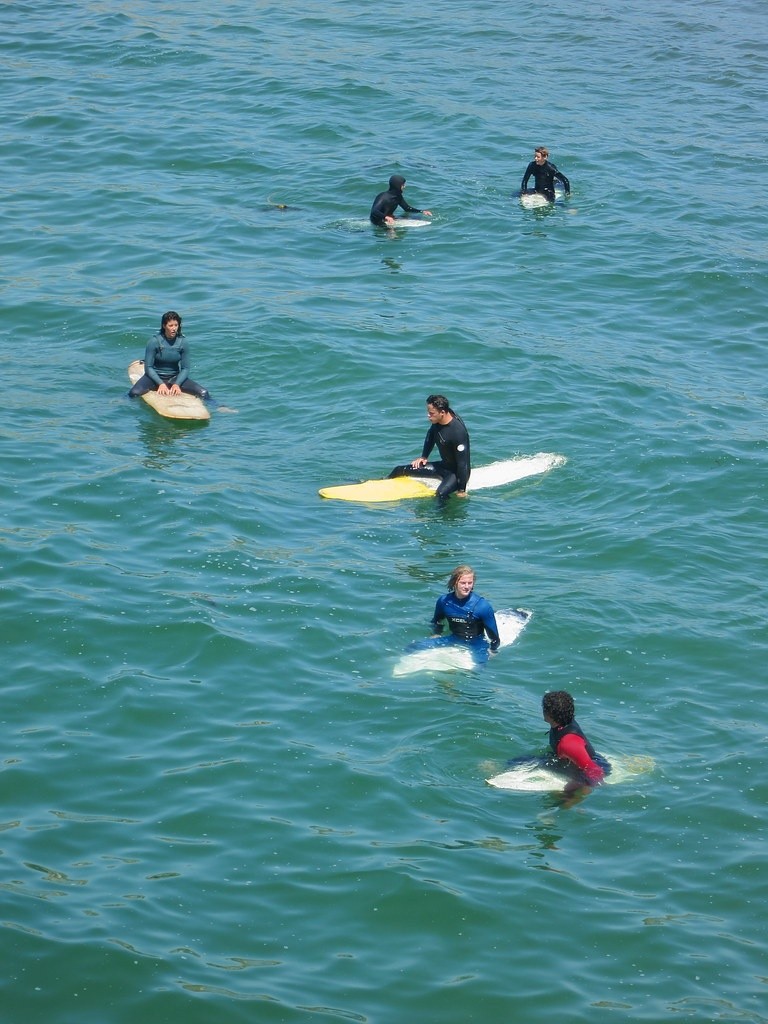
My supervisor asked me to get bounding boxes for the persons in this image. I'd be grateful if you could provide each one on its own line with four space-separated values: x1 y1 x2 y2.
513 146 576 214
370 175 432 238
403 565 501 670
360 395 470 504
127 312 239 414
480 692 612 827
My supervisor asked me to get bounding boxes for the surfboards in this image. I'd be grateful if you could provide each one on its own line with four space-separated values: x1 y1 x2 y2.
128 359 211 421
318 451 567 503
520 190 561 210
385 218 432 229
393 607 532 680
484 754 656 793
